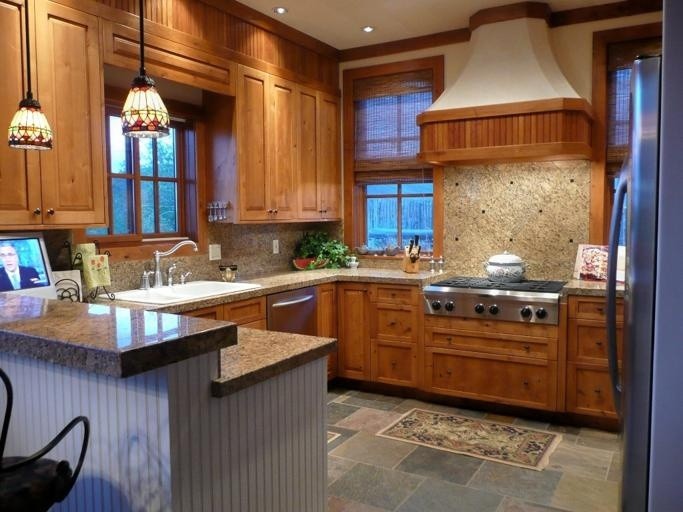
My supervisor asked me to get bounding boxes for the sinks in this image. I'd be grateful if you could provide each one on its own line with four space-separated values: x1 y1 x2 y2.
99 280 261 305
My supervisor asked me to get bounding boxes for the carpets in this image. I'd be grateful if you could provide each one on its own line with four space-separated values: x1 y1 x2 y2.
375 407 563 472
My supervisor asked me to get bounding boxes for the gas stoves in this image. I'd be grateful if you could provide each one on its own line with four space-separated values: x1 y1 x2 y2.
424 277 567 326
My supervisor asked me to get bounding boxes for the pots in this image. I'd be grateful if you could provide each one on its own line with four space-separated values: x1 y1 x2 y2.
482 249 526 284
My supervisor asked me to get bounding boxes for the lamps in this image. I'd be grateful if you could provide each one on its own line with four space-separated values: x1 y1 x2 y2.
120 0 169 138
7 0 52 150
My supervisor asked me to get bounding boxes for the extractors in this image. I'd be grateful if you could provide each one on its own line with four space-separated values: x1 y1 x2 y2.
415 5 597 169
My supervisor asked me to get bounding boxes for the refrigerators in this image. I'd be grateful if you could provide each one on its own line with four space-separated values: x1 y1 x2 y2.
607 0 683 511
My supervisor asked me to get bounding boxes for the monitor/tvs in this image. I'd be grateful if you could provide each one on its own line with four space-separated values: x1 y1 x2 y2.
0 232 58 300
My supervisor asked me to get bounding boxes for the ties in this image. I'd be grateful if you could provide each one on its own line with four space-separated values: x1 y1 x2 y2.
11 272 21 291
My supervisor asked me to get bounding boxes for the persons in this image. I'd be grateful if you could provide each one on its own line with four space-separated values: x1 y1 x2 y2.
1 244 42 289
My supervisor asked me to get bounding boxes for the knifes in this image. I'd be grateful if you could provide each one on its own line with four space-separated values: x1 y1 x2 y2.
414 235 418 248
416 246 421 256
408 239 414 254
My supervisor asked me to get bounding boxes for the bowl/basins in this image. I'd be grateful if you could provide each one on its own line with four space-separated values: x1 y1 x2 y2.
368 251 376 255
376 251 386 256
350 262 359 268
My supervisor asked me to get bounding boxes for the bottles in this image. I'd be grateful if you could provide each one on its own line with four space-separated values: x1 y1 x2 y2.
429 255 444 273
350 254 357 262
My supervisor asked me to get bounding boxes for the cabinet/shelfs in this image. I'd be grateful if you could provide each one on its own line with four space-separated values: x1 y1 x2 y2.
337 283 624 430
2 1 111 229
177 282 335 381
204 63 342 225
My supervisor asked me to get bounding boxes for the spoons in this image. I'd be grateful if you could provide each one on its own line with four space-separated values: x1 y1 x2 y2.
208 202 227 223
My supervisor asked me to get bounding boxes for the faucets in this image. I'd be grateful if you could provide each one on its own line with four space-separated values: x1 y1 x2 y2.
152 240 198 287
